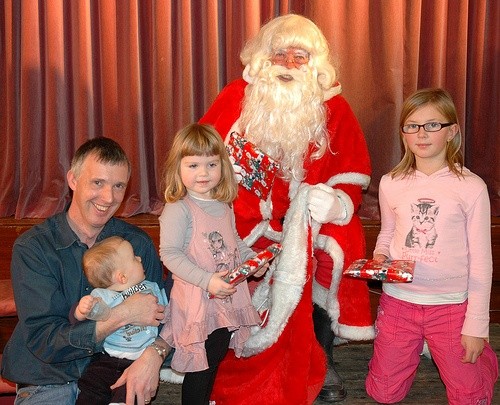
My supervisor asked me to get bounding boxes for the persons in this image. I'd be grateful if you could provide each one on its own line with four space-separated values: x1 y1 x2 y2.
181 11 376 405
0 135 187 405
364 86 499 404
66 237 173 405
156 121 271 405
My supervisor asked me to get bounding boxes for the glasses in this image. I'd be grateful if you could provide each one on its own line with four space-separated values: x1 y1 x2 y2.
401 121 455 134
271 51 308 64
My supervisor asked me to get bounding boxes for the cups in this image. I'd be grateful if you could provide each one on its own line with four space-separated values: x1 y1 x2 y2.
85 297 111 322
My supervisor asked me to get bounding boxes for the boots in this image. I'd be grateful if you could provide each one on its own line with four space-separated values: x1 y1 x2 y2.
312 302 347 402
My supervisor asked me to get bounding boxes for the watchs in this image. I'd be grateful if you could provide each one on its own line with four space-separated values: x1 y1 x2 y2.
150 342 167 361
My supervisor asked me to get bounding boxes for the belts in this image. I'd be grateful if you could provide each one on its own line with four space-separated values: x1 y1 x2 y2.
17 384 37 390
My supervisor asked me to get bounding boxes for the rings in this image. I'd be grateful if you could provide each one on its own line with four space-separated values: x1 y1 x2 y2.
145 399 151 402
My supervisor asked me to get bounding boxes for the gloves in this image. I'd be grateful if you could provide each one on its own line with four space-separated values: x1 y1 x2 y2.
306 182 347 224
